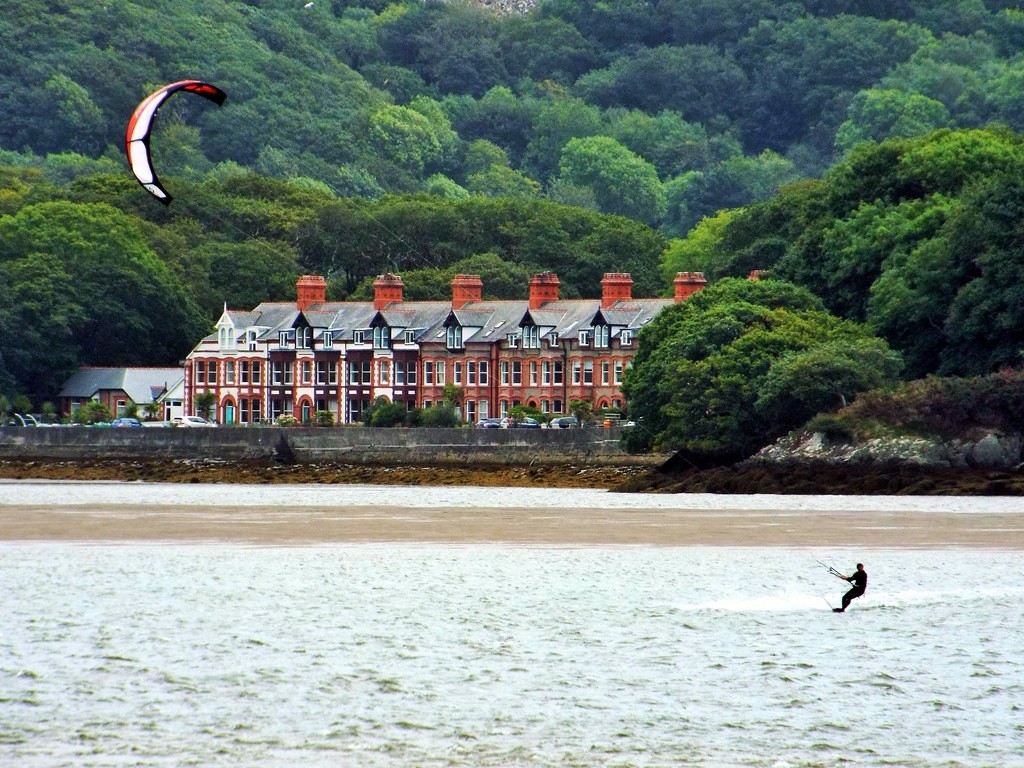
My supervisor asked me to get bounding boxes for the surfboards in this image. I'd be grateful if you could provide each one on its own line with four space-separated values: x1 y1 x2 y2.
833 608 843 612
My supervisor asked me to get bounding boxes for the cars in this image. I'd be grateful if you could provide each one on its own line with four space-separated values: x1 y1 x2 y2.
475 417 542 428
111 418 146 428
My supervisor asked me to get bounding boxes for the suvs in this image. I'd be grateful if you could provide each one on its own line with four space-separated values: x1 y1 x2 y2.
172 416 218 428
549 416 576 428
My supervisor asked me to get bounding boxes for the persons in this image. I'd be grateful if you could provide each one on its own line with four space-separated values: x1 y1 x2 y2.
833 563 868 612
